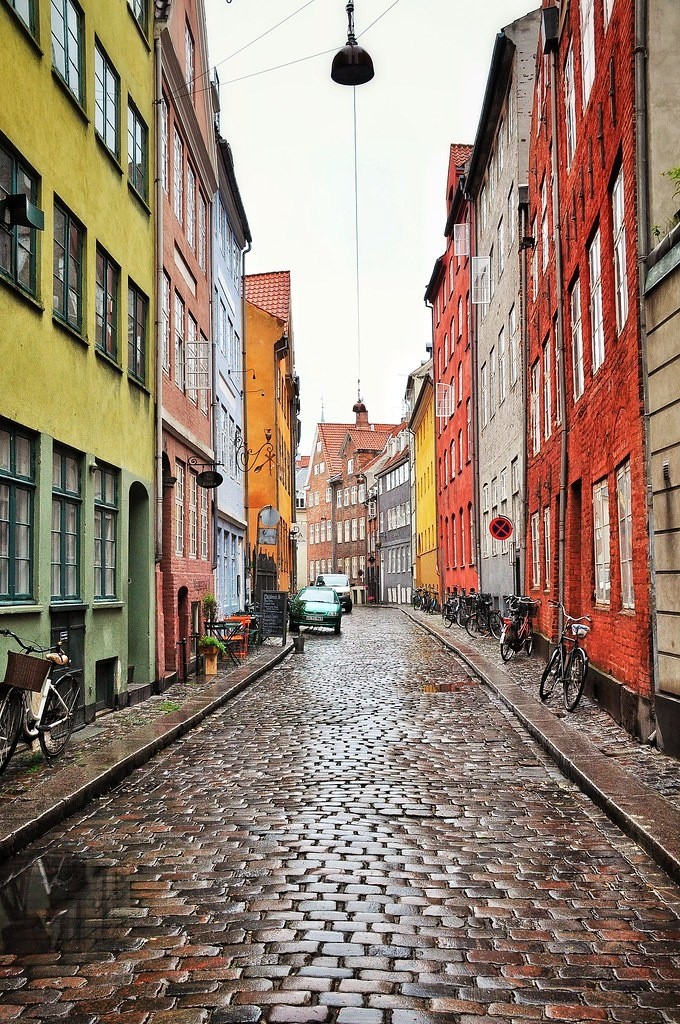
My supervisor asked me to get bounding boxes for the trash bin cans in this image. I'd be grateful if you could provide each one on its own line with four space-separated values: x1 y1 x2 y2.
293 637 304 653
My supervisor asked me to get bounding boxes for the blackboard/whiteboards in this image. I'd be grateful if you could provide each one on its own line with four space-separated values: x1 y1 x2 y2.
259 590 288 637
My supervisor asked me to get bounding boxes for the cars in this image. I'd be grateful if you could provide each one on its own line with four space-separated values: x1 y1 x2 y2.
290 585 345 635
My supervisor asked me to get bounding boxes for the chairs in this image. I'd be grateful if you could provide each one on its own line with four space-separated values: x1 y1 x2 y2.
213 610 265 659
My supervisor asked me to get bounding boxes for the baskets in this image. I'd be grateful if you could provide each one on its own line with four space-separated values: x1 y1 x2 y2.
465 597 473 606
520 606 538 617
3 650 52 693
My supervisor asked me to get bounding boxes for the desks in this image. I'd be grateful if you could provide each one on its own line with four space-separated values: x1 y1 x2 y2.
204 620 242 667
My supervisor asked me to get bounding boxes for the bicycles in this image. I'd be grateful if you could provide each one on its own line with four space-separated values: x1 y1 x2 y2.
0 629 84 778
412 589 542 662
540 599 594 711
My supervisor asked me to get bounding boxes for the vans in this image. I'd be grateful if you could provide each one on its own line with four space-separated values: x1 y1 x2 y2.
317 574 353 613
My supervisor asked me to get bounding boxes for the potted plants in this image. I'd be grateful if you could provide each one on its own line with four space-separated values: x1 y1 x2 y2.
658 166 680 221
198 593 226 676
290 597 306 653
